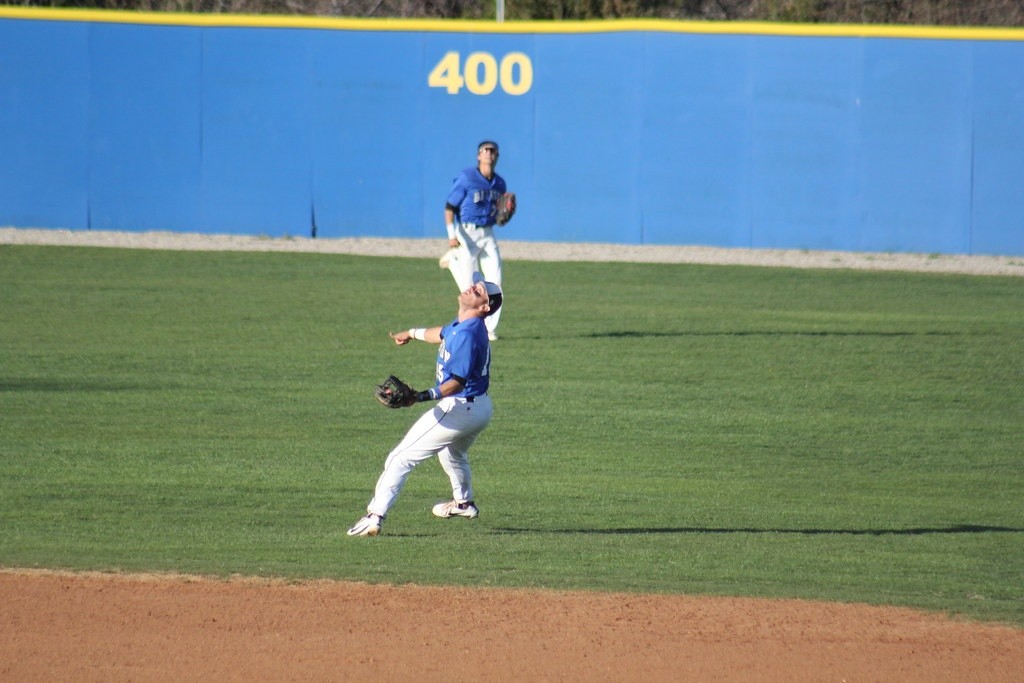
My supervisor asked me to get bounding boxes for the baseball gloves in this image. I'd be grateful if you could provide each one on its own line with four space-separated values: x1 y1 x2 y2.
375 374 419 409
495 192 516 226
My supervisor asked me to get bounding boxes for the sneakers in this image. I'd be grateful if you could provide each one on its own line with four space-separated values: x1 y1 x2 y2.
432 500 479 519
348 515 381 536
489 333 498 341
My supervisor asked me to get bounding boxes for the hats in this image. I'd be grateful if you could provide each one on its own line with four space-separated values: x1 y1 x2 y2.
473 271 502 316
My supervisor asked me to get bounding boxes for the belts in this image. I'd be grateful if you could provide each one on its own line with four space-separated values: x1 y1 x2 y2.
467 392 487 402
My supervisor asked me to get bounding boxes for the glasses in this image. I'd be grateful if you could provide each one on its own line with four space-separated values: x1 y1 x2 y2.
478 148 497 154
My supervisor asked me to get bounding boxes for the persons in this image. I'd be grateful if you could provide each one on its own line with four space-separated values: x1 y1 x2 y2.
444 140 516 341
348 280 504 538
